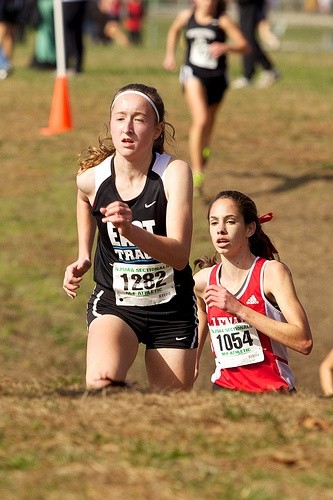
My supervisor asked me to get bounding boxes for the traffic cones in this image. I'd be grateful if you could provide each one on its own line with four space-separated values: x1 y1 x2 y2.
39 73 77 137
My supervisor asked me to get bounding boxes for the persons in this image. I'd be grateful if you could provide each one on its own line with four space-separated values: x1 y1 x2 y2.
62 84 200 393
319 349 333 398
192 190 312 393
163 0 250 190
1 0 280 90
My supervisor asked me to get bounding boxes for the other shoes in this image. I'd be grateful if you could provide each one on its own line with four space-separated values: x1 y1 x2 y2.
202 144 210 165
232 76 250 87
258 68 283 90
193 171 204 196
